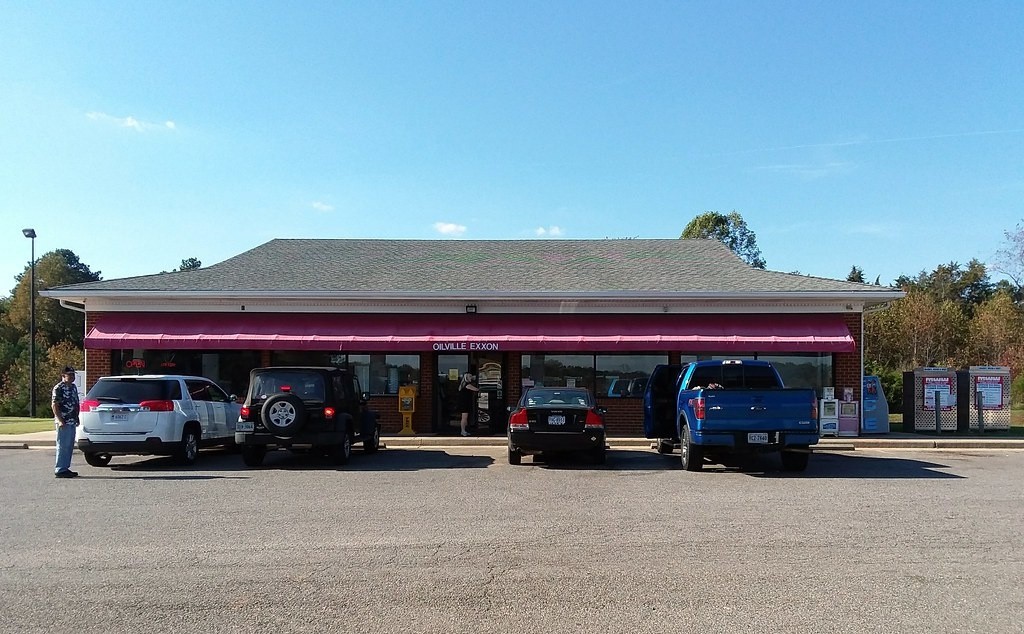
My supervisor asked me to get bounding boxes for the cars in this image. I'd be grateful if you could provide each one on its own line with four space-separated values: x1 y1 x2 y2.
506 386 609 465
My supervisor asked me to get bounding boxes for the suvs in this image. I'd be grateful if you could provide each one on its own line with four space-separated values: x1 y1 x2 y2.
76 372 243 467
234 366 380 468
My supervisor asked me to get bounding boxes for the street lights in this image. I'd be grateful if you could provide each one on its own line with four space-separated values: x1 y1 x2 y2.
22 228 37 418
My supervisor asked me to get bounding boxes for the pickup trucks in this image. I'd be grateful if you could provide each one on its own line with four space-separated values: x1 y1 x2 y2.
607 377 649 398
642 358 820 473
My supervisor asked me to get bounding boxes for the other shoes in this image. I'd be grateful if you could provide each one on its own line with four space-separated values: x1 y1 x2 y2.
461 431 471 436
55 469 78 478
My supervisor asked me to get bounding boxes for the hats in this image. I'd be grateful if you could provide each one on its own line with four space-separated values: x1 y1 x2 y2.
62 366 77 373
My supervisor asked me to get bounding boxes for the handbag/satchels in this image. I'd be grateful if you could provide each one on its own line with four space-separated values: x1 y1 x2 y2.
455 383 474 409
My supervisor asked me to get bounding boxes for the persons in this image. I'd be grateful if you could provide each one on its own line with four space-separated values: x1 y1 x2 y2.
52 366 80 478
458 374 479 436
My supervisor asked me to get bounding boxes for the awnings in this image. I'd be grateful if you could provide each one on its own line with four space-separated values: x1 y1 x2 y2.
84 312 855 351
